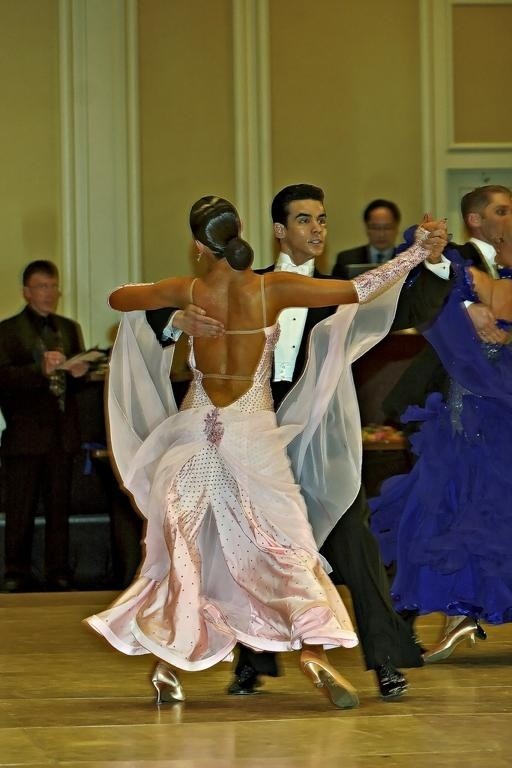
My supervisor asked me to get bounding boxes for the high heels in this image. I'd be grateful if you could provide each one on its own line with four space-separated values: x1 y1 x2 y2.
420 615 477 662
151 664 186 706
299 650 360 709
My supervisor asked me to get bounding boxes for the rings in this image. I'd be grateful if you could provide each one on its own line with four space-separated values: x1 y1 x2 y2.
56 359 60 365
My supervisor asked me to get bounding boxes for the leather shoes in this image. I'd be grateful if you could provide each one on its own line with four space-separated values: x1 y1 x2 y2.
228 666 260 694
375 655 410 698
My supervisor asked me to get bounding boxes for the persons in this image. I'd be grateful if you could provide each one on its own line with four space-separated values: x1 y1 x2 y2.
97 315 142 589
331 197 403 279
1 258 107 592
102 191 447 712
142 182 455 701
